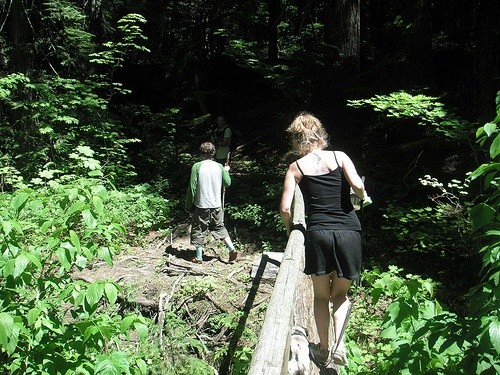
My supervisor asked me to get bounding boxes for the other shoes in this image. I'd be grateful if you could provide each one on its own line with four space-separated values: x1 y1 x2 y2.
192 256 203 264
229 249 237 261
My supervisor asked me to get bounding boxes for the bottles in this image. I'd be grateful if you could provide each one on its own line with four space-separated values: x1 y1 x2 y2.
349 186 361 210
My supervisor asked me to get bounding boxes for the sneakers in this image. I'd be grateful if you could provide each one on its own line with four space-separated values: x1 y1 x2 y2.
331 342 348 366
309 342 330 364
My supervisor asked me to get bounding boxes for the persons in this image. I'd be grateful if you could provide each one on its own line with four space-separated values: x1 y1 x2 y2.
278 114 367 366
185 142 237 263
